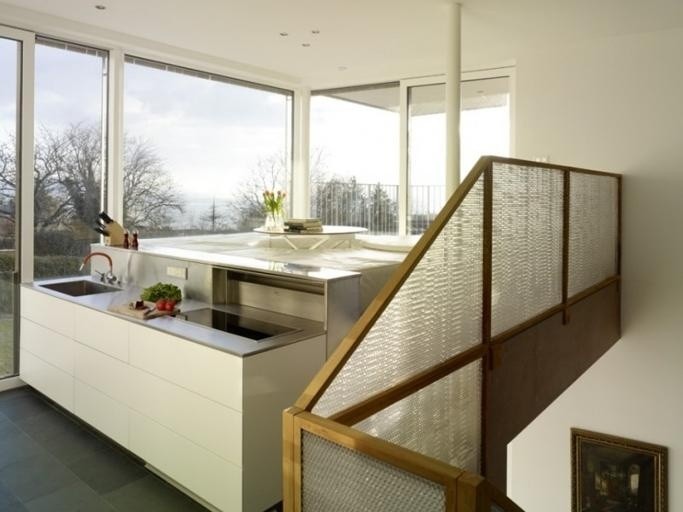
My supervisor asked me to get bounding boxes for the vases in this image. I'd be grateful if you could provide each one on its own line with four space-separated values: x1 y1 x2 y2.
264 212 284 233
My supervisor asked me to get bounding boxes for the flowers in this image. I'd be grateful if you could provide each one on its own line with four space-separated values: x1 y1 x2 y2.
263 191 286 222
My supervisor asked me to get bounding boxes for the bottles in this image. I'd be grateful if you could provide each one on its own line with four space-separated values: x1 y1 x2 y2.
121 227 140 251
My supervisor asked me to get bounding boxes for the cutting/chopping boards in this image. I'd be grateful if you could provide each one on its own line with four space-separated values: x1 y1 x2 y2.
106 302 178 321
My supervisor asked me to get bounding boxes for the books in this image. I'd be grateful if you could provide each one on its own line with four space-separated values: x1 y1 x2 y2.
283 218 324 234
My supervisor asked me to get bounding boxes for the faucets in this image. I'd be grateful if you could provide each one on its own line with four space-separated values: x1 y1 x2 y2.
78 253 116 285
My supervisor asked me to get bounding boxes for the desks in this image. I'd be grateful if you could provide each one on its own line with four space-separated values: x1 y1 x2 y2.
253 225 368 250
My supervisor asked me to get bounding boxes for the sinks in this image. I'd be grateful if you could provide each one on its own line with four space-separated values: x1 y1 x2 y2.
40 279 122 297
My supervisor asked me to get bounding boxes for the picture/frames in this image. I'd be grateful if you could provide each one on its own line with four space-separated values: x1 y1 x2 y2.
570 428 667 512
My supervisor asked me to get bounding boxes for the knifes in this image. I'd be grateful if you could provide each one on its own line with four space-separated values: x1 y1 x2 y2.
143 306 156 316
93 210 114 237
